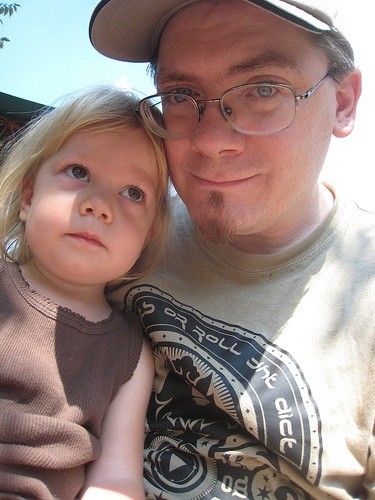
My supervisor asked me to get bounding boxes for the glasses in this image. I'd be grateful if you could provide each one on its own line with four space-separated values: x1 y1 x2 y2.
135 64 340 140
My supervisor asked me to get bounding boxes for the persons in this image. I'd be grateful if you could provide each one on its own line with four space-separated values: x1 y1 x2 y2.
89 0 375 500
1 86 174 499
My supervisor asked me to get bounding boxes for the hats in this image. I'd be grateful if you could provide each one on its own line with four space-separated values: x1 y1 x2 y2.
88 0 356 62
